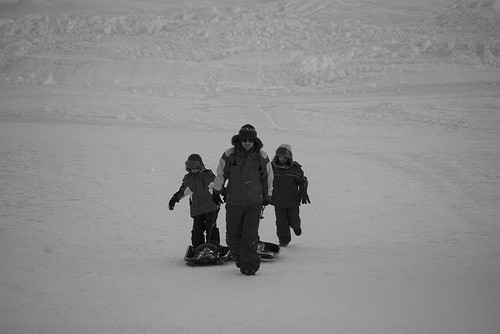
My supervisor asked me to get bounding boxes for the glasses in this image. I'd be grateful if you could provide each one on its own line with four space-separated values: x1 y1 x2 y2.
242 138 254 143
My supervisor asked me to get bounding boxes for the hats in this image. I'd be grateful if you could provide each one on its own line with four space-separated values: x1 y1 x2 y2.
275 144 293 164
185 154 205 174
239 124 257 138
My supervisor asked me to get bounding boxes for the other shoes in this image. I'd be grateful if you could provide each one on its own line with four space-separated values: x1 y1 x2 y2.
293 223 301 236
279 240 288 246
235 255 255 275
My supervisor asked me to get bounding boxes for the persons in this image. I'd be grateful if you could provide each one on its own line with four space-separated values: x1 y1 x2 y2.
211 124 274 275
169 154 226 257
269 144 312 247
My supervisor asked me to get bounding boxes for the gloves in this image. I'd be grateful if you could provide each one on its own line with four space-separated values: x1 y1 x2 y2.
212 190 223 205
222 191 227 202
262 196 270 205
298 191 310 204
169 197 177 210
